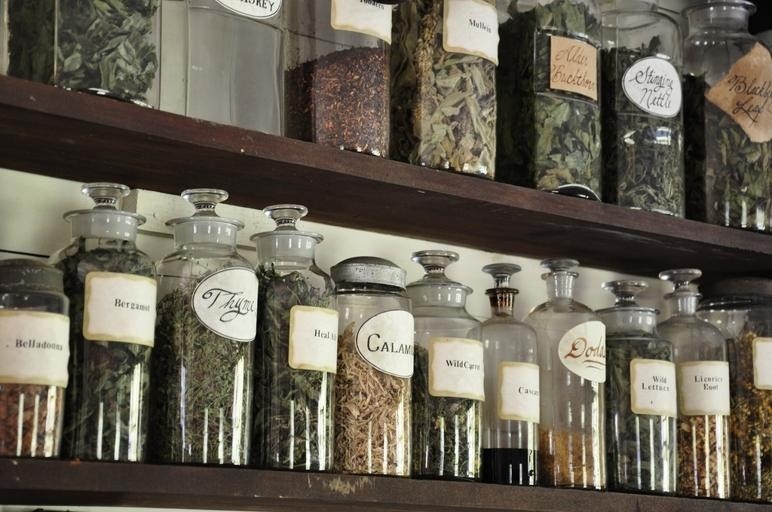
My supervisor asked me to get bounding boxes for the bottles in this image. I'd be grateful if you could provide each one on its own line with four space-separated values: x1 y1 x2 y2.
518 258 607 492
184 1 290 138
148 188 259 468
497 1 601 200
404 250 482 480
690 291 772 503
390 2 500 181
282 0 395 161
0 257 70 459
677 1 771 235
248 203 337 474
0 0 168 111
330 255 414 480
655 267 733 502
48 182 160 462
466 262 540 487
597 1 688 221
594 277 681 497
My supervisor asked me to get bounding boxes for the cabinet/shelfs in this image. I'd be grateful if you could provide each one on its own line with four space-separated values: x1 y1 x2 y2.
0 74 772 512
